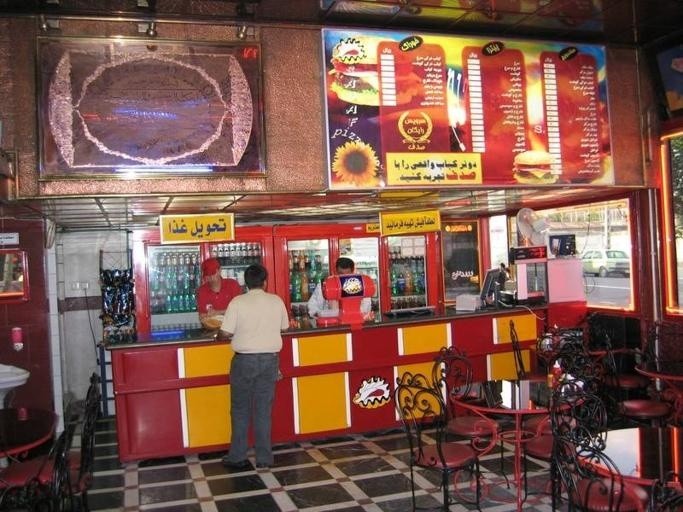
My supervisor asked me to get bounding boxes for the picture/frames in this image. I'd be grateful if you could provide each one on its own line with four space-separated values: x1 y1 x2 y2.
33 34 267 183
643 31 682 122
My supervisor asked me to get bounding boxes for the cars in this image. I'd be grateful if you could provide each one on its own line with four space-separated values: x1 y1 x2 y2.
582 249 630 278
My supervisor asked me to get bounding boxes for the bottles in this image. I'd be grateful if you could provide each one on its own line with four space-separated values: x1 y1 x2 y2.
391 269 425 296
151 322 203 342
211 243 262 265
389 247 424 268
153 251 200 266
356 270 378 297
392 297 426 308
371 299 380 322
289 248 323 271
290 272 325 302
292 304 310 330
149 265 201 290
150 290 198 313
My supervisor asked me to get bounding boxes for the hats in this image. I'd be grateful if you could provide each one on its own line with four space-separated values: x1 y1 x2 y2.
201 258 219 278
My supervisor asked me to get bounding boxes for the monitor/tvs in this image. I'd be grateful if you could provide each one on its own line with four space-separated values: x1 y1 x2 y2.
480 268 504 310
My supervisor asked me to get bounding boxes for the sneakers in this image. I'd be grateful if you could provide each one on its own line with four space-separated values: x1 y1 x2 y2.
222 457 249 468
256 462 271 472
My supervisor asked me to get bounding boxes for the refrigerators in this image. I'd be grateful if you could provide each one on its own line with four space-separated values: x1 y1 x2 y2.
134 225 275 342
383 223 439 318
279 220 383 331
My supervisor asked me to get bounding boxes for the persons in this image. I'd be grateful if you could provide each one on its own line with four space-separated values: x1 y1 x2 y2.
219 264 289 473
307 256 371 320
197 259 242 327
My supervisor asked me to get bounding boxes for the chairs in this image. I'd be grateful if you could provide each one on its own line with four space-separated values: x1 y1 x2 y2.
0 374 107 512
392 312 682 512
